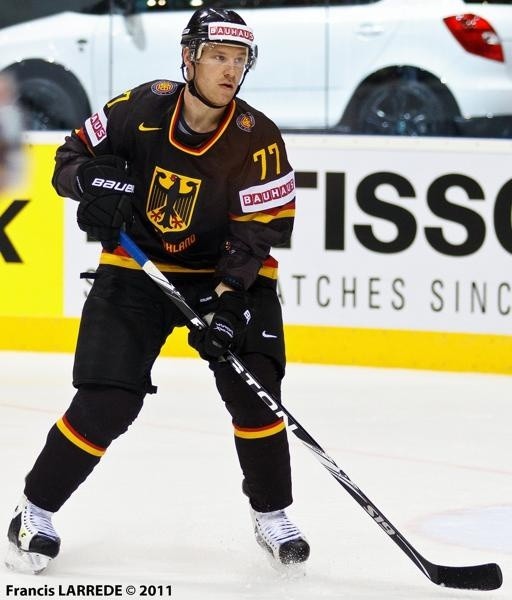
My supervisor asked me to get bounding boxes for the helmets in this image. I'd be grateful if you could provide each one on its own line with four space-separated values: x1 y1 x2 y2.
180 7 256 64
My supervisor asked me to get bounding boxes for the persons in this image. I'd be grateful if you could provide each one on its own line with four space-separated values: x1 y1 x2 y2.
1 6 312 574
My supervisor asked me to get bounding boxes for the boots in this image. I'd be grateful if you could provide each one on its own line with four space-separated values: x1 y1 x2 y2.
252 508 310 564
7 495 61 559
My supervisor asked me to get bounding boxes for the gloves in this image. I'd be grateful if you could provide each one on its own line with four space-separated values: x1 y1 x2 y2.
188 290 252 371
76 154 133 241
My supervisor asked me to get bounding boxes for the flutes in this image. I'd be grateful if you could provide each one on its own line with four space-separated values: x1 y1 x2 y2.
116 230 502 590
